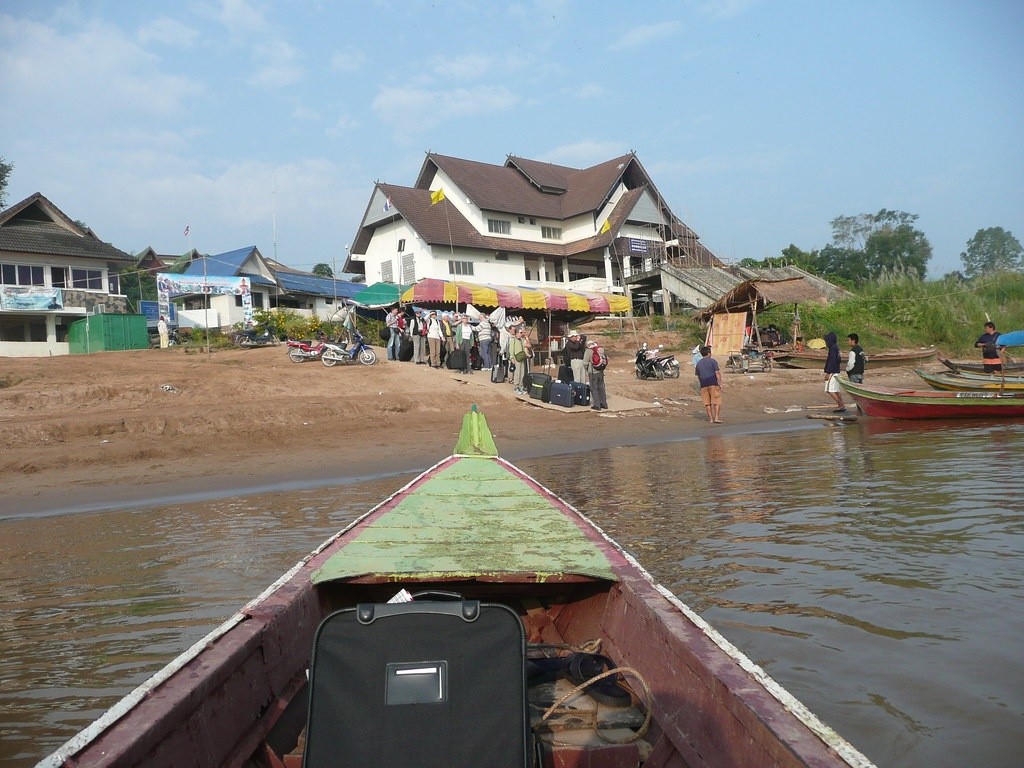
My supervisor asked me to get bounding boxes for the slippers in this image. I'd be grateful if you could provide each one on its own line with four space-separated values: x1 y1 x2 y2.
560 651 632 709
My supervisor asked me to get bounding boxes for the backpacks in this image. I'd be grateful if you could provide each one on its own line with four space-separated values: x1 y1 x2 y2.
591 346 608 370
542 380 553 404
550 381 576 407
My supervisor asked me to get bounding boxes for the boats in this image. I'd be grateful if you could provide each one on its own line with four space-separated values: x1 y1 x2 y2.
913 367 1024 392
935 354 1024 375
834 374 1024 421
772 344 939 371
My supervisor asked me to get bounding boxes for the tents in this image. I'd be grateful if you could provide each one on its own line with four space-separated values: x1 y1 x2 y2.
346 277 640 366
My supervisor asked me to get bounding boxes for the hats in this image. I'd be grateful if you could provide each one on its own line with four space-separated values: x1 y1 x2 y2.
566 330 580 337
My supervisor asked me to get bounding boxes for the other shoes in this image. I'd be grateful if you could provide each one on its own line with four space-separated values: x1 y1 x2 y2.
600 403 608 408
481 367 491 371
590 406 600 410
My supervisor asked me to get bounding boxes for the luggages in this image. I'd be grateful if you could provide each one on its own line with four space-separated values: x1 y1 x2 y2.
446 349 467 369
490 353 505 383
398 341 416 362
569 381 591 406
557 365 574 384
301 589 534 768
523 358 536 393
530 357 551 402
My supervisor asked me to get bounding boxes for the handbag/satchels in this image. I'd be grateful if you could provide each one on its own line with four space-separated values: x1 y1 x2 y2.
379 327 391 341
514 351 526 362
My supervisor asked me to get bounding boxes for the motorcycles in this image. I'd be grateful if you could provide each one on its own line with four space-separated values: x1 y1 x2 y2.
285 325 336 364
320 326 377 367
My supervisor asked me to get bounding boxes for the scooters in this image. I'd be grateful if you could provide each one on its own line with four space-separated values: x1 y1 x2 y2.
237 319 281 351
634 341 681 380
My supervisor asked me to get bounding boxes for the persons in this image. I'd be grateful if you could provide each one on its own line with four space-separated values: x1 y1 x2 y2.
502 316 534 394
48 297 61 309
823 332 847 412
410 309 500 374
695 346 724 423
845 333 864 416
567 329 587 384
158 316 169 348
385 306 407 360
750 323 786 347
975 322 1005 374
583 340 608 410
562 338 571 368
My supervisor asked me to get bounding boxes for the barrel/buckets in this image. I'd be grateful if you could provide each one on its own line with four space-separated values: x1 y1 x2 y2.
561 336 568 350
551 339 558 351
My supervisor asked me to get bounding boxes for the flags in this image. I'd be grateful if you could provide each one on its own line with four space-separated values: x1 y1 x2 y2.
184 225 189 236
601 220 611 236
383 199 390 212
431 188 444 204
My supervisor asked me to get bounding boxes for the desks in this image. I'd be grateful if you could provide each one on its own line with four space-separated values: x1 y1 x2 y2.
534 350 563 366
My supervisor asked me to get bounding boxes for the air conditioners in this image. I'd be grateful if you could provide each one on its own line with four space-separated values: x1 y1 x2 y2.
93 304 105 314
109 283 114 291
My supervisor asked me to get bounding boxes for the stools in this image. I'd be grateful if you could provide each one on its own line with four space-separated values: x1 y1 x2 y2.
556 356 565 366
